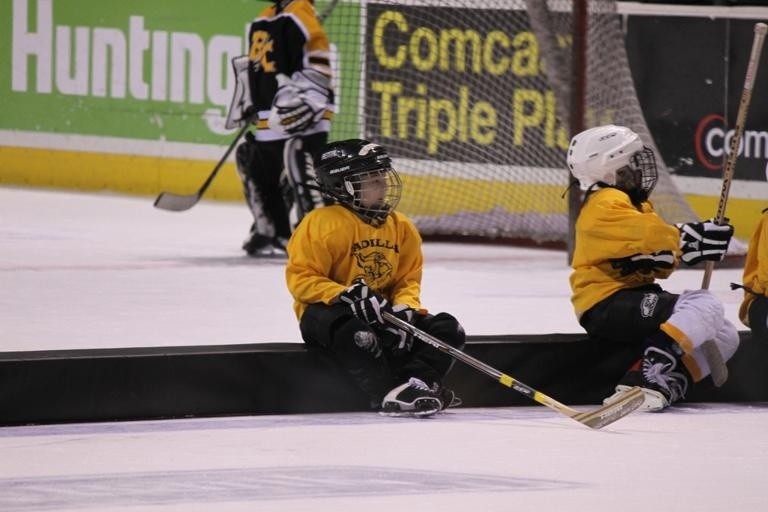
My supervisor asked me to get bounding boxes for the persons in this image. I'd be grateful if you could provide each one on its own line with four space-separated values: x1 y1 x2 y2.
236 0 335 257
739 208 768 332
285 139 467 416
568 123 740 412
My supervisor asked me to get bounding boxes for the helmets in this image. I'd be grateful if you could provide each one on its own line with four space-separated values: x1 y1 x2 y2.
566 125 658 204
313 140 404 227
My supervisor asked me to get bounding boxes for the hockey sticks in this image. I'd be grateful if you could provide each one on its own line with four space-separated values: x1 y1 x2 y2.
381 311 644 428
154 0 337 212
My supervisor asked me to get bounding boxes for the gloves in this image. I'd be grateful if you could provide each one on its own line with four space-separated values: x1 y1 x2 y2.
674 218 734 264
272 67 332 134
335 275 415 358
610 250 674 279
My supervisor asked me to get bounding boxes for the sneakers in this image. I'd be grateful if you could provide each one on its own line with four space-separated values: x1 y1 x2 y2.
243 233 294 258
616 346 695 406
380 369 455 418
